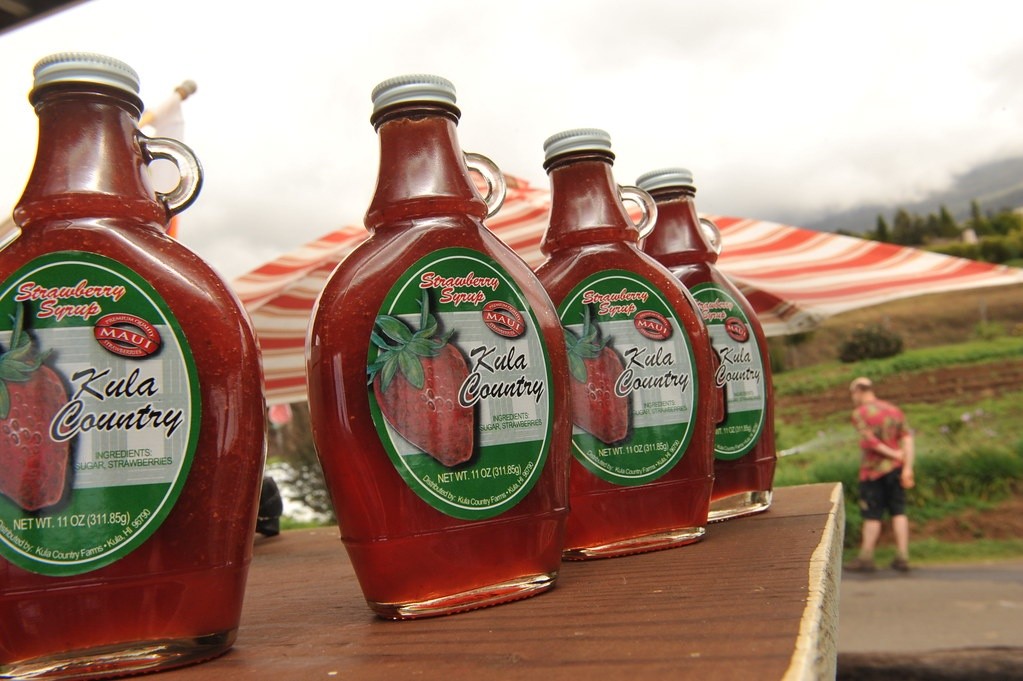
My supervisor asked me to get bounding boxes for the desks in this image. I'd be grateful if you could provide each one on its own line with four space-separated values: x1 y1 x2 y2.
101 480 848 681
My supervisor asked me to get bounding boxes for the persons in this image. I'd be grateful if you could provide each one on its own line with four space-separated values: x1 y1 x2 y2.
844 378 915 573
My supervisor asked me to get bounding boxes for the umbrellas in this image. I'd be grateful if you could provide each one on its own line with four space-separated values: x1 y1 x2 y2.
227 170 1023 401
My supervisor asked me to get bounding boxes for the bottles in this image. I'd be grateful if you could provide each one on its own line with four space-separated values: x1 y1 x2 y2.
633 167 778 524
0 50 268 679
533 128 716 563
306 74 573 622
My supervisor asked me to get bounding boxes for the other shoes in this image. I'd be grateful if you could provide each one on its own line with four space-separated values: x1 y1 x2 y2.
890 559 912 574
842 560 875 572
